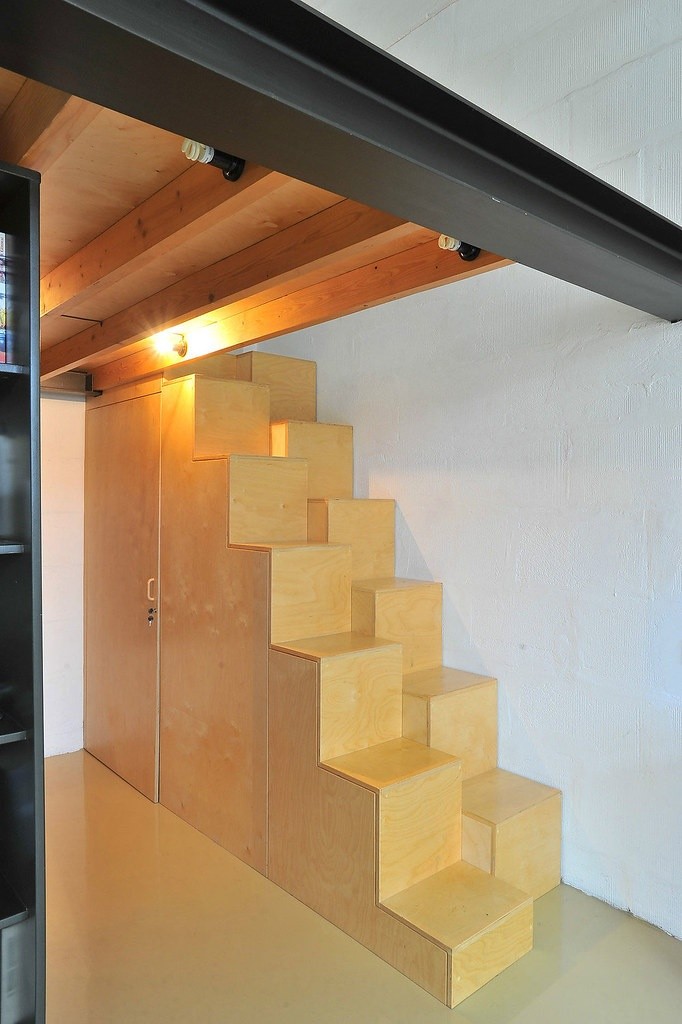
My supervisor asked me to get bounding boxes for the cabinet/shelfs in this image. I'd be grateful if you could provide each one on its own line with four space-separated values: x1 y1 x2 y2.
0 161 70 1024
